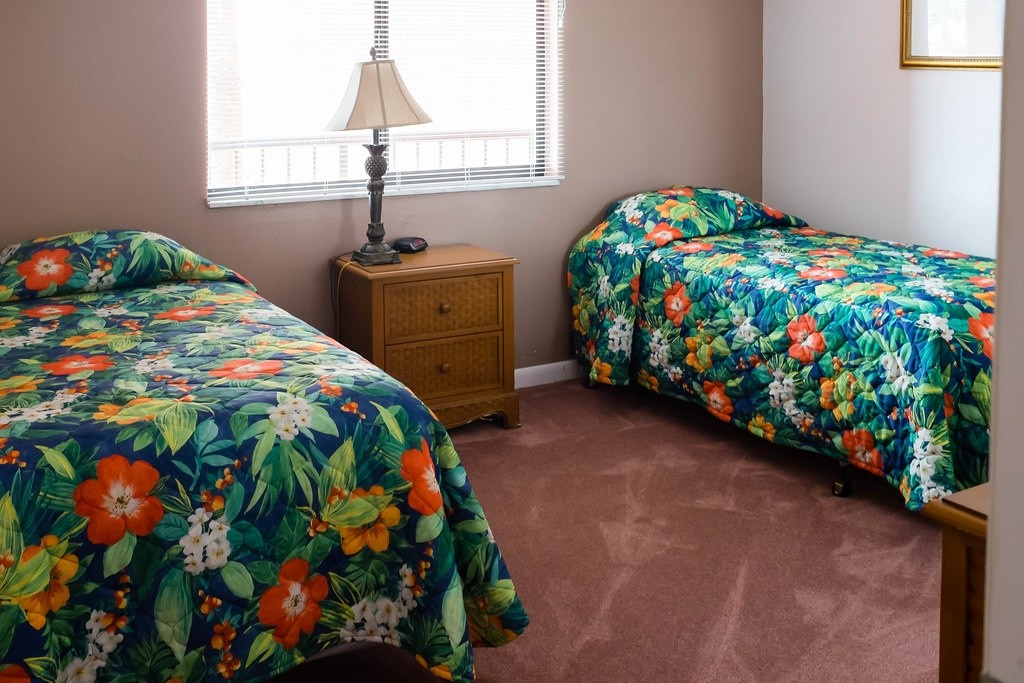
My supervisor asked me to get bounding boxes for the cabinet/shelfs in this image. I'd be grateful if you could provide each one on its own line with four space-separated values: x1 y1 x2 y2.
921 482 989 683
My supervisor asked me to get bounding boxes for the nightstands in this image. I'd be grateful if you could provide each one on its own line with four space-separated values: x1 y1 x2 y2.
329 242 522 430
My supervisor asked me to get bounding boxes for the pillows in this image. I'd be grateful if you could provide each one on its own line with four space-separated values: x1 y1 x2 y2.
0 229 259 305
603 186 785 249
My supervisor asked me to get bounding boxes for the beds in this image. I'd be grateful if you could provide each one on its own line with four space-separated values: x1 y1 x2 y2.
566 213 996 511
0 279 528 683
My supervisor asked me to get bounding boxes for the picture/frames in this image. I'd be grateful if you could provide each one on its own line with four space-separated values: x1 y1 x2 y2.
899 0 1006 72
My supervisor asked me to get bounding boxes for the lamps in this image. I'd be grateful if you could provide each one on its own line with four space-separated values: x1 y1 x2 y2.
324 46 434 267
